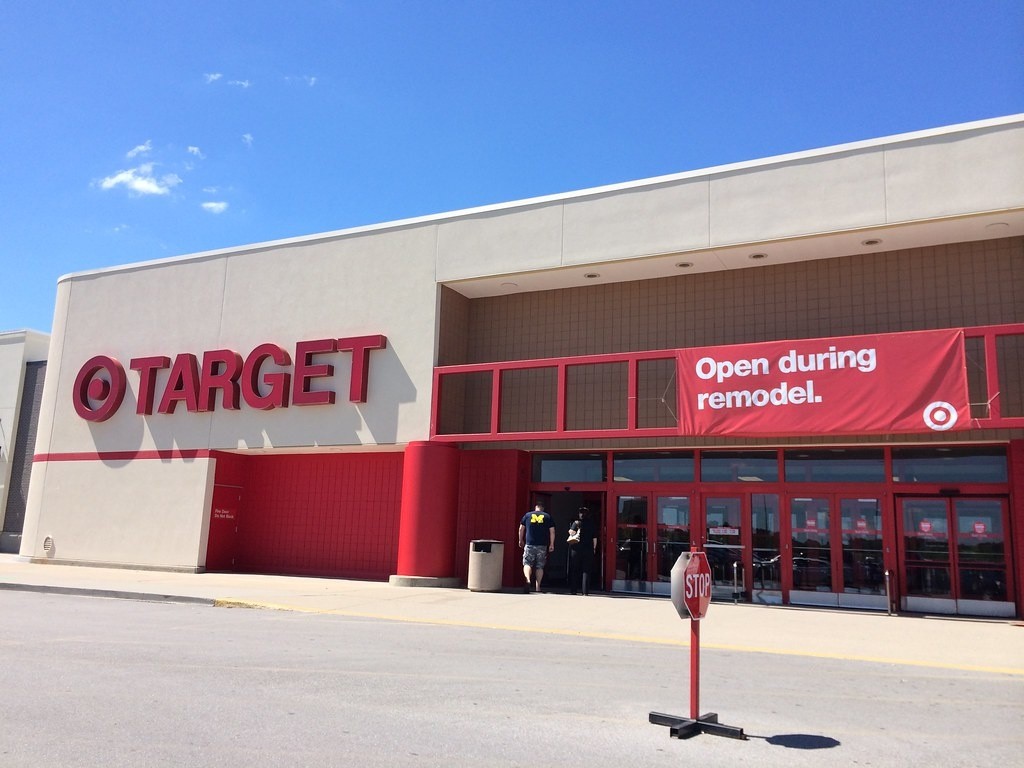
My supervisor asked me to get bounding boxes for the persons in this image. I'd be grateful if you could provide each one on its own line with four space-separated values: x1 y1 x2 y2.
518 502 555 592
568 505 597 596
624 515 647 578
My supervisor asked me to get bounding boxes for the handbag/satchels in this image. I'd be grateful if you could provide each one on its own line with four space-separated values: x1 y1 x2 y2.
567 521 581 545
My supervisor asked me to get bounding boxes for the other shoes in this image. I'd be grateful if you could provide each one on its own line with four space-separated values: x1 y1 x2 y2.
524 581 530 594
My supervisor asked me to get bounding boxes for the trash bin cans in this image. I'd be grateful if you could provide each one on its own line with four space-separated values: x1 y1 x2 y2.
466 539 505 592
